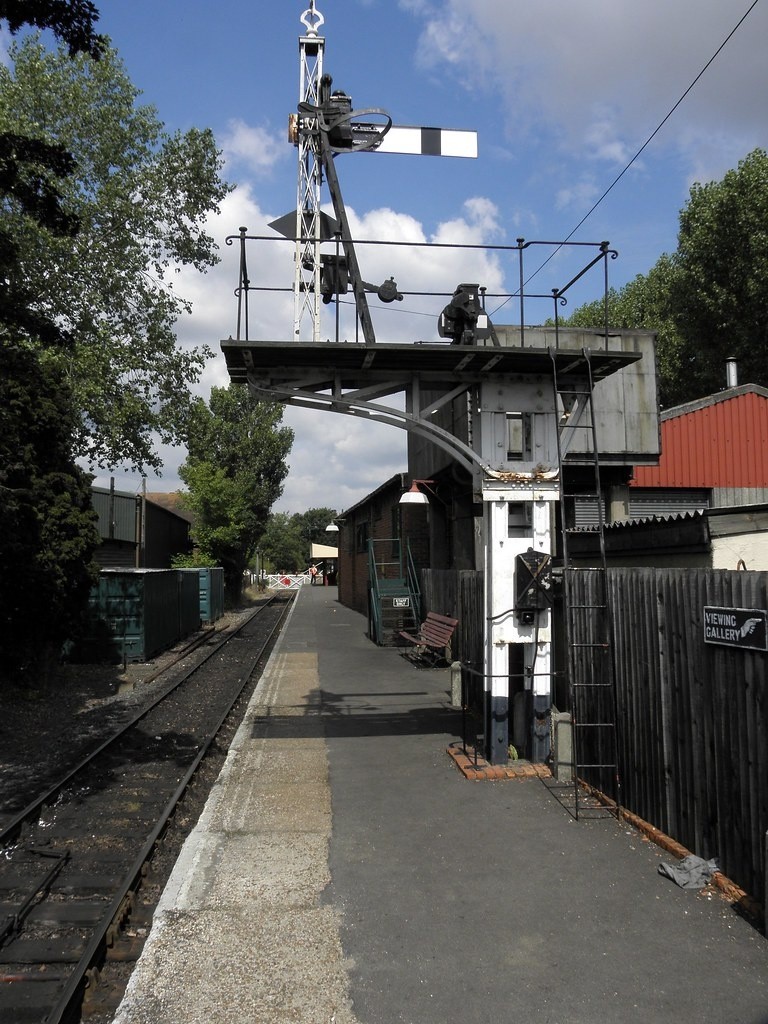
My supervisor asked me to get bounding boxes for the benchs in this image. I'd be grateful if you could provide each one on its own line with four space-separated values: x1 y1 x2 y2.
399 611 459 669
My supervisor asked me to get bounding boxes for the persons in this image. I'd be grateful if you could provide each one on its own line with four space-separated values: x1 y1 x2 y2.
310 564 317 584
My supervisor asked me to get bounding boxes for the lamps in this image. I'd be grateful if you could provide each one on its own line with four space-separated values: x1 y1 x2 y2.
399 477 436 504
